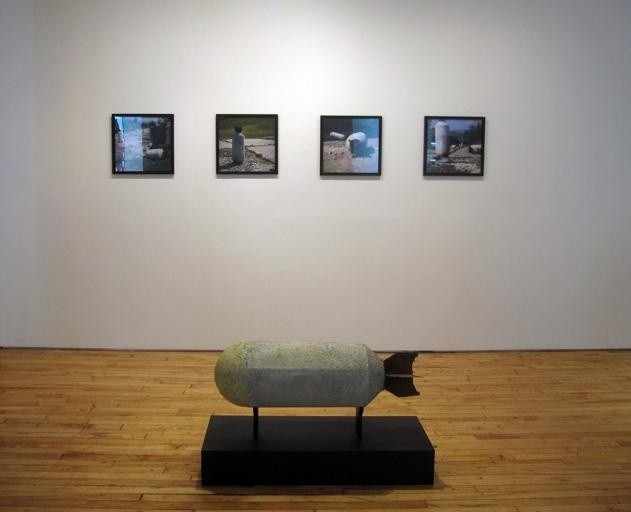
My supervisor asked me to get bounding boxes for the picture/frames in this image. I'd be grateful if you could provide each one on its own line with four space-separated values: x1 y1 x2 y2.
320 114 381 177
111 114 174 175
215 113 278 175
423 115 484 177
